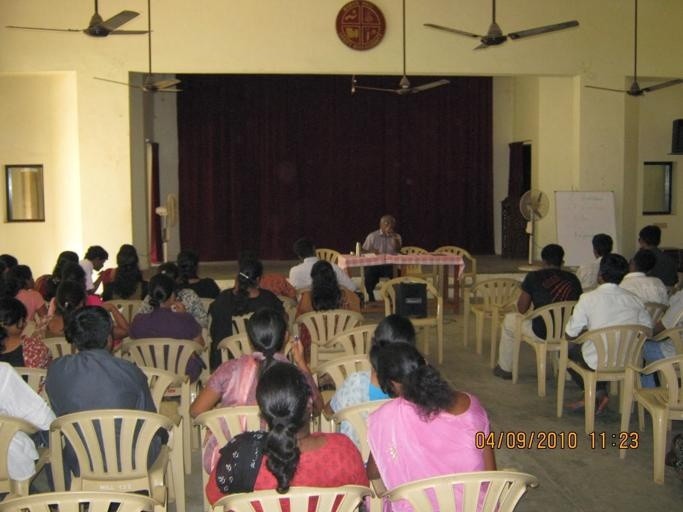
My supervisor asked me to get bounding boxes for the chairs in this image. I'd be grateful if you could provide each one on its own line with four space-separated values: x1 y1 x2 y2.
315 248 342 266
432 246 477 313
401 246 435 283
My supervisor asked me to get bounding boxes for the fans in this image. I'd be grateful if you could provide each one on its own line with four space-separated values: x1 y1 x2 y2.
6 0 153 37
352 0 451 95
584 0 683 95
155 193 177 264
423 0 579 50
519 189 549 271
93 0 184 93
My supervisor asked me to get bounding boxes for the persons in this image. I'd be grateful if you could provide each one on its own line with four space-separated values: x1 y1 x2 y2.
618 249 669 321
1 244 496 512
361 214 402 303
493 244 583 380
641 289 682 389
574 233 614 290
637 225 679 287
563 253 654 416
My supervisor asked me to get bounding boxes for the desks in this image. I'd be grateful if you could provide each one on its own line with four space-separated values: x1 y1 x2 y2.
337 252 465 313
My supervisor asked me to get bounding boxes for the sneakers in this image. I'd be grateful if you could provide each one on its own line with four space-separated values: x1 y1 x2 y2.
492 364 512 379
563 392 611 416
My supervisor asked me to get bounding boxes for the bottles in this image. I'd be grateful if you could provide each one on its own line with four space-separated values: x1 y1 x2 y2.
356 241 360 257
